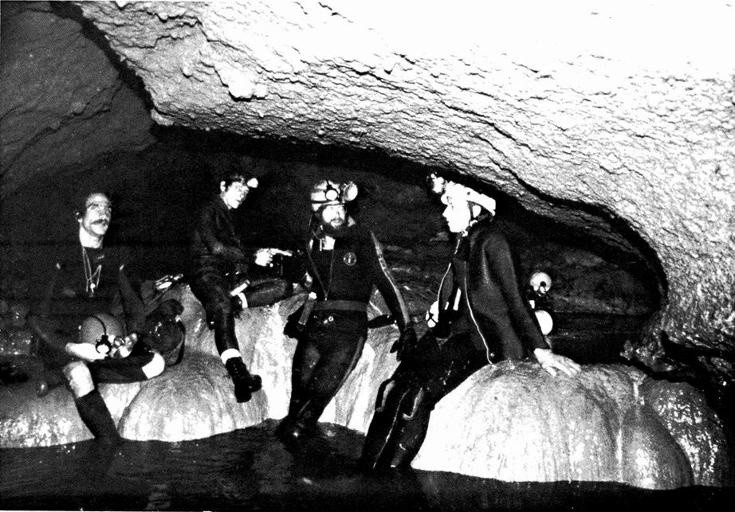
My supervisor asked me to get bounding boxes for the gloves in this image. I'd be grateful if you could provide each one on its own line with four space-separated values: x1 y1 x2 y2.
388 321 419 361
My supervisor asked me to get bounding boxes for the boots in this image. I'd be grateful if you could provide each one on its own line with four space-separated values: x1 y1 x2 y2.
225 353 265 404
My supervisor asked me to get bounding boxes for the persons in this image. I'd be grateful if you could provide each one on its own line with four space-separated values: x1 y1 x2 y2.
223 173 421 500
301 168 584 497
190 167 295 403
28 182 167 478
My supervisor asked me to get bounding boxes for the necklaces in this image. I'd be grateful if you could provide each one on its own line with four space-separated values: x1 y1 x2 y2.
81 247 99 292
83 249 102 293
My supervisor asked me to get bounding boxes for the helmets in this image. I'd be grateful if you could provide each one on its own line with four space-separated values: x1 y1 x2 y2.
308 176 354 215
77 312 126 357
